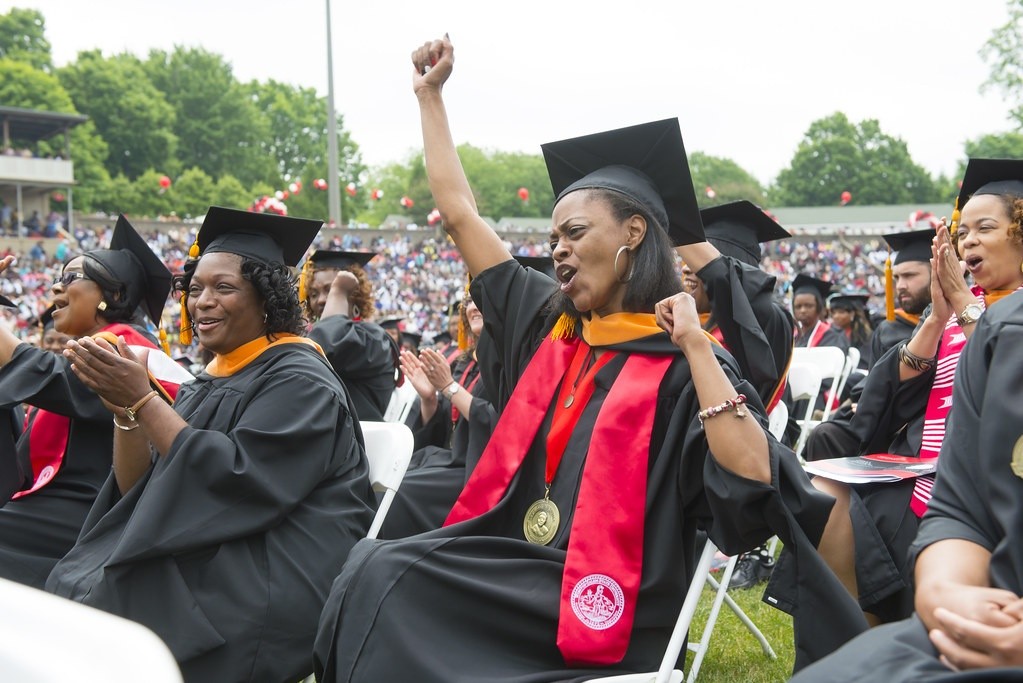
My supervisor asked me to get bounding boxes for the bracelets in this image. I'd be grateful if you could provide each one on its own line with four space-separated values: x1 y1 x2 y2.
898 344 934 371
697 394 746 430
440 381 454 392
113 418 139 430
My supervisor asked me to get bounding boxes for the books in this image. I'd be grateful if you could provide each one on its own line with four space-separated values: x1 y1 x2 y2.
801 453 937 483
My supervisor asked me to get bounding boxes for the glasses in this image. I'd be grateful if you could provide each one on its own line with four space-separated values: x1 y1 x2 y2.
52 272 93 287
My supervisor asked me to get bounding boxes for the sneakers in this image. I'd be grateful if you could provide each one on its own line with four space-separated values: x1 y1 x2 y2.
706 550 735 572
726 553 776 591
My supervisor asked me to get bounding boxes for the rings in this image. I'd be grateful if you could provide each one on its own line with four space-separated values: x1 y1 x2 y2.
430 367 434 371
936 249 949 256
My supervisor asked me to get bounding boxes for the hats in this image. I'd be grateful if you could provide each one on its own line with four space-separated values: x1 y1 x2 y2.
373 317 403 330
297 250 377 310
949 158 1023 236
457 255 559 350
791 273 835 300
81 214 173 356
40 303 56 328
540 117 707 341
179 204 324 347
442 300 462 316
432 332 453 345
400 332 421 348
699 200 793 262
828 295 869 311
879 227 952 323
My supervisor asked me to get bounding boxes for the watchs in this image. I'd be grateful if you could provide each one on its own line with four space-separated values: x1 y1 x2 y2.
125 389 159 421
446 383 459 401
956 303 984 327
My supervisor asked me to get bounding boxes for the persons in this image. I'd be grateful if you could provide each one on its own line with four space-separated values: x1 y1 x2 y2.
0 205 114 441
814 159 1023 625
785 194 1023 683
707 228 939 591
676 200 801 413
313 31 870 683
300 249 405 421
377 270 497 540
0 215 198 591
313 215 553 364
145 212 205 373
43 206 379 683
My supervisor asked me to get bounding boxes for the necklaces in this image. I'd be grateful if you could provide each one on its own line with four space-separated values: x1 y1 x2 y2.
565 347 593 408
979 283 1023 311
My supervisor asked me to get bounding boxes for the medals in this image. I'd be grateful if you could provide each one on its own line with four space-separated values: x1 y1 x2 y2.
524 498 560 545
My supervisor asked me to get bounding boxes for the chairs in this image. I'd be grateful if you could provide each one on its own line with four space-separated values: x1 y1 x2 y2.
768 347 860 558
583 400 790 683
358 420 414 538
